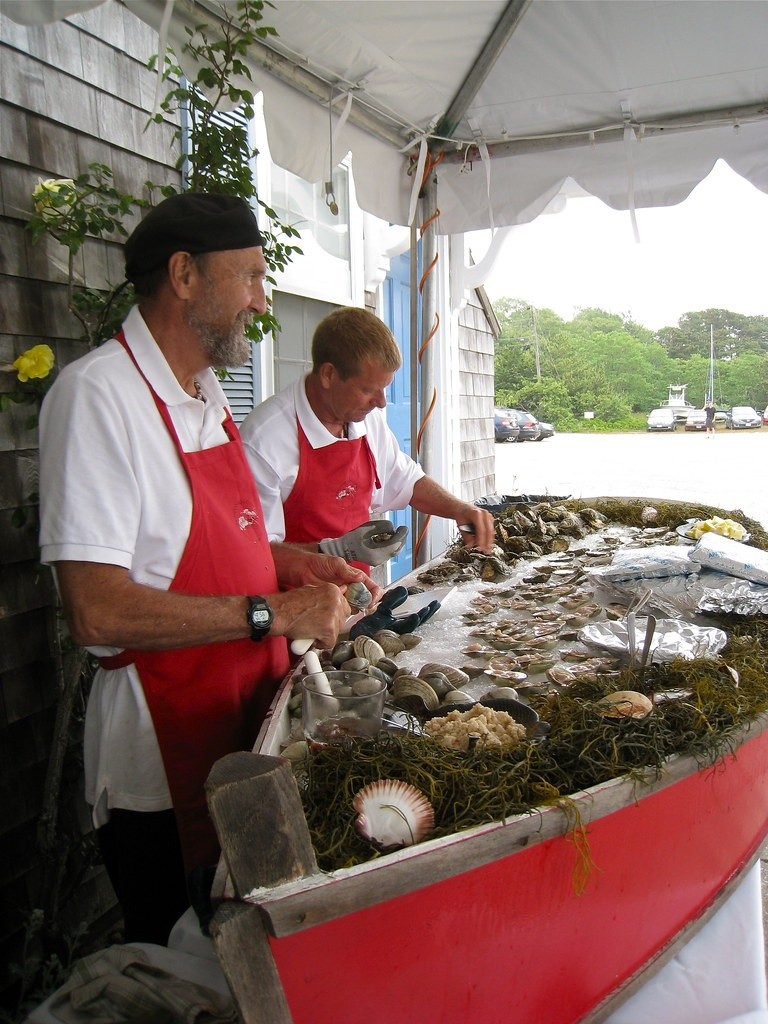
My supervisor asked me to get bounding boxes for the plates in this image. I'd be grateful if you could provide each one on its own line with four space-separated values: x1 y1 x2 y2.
677 522 752 543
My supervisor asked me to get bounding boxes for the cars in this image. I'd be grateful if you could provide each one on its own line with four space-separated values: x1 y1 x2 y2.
540 420 555 439
495 411 520 442
506 408 540 441
684 409 715 431
724 405 762 429
645 407 678 432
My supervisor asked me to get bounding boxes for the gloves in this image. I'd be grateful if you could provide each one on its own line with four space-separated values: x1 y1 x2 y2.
319 519 409 567
346 585 441 640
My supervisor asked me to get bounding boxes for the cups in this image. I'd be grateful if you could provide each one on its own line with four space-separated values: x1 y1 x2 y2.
303 669 388 755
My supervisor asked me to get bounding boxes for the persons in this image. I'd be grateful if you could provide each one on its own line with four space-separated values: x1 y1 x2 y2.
238 308 495 657
702 401 717 439
41 195 377 944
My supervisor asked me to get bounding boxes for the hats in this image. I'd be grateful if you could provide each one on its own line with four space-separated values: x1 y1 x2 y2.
123 192 266 286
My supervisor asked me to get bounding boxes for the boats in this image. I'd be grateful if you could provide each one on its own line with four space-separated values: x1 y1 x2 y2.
208 492 766 1024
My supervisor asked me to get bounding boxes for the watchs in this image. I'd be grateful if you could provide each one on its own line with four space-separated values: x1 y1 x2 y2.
245 596 273 642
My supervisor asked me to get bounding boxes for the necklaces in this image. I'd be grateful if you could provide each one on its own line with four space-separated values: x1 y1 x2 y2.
342 424 349 438
194 377 204 400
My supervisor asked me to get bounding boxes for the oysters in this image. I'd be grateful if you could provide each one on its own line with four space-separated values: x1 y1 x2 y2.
418 495 609 585
